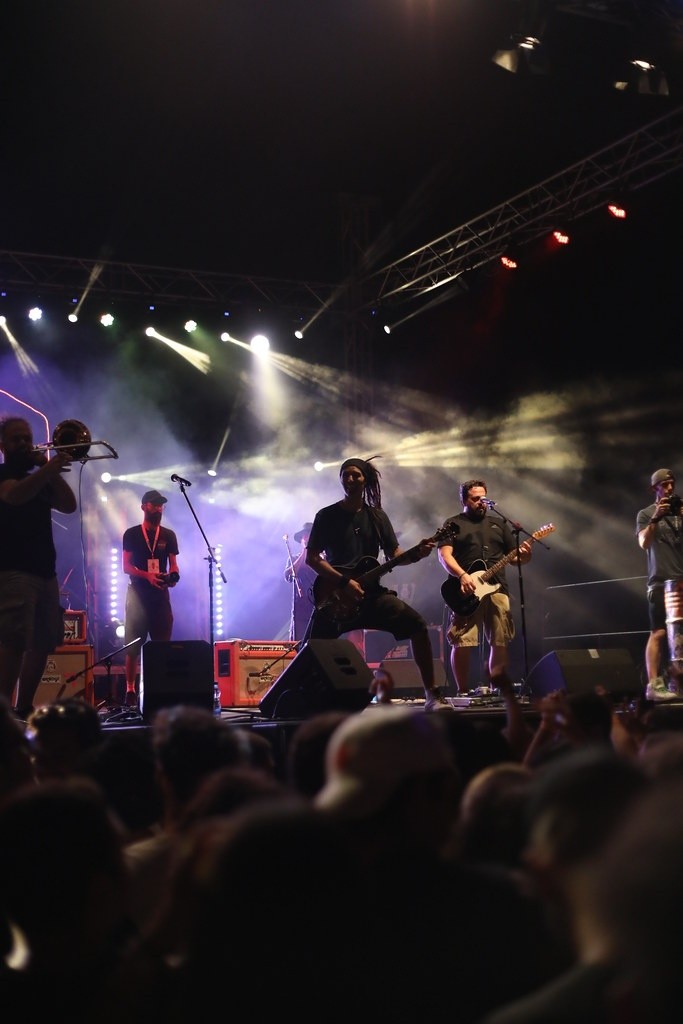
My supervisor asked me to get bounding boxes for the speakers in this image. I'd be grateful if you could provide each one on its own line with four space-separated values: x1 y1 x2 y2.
11 644 94 710
140 640 214 722
519 648 642 705
379 659 446 698
213 640 299 708
260 638 378 722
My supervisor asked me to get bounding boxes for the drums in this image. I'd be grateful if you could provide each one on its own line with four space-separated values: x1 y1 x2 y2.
663 578 683 699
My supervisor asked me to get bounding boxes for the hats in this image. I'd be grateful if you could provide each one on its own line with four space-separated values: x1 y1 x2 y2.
141 490 167 505
650 468 674 487
294 523 313 544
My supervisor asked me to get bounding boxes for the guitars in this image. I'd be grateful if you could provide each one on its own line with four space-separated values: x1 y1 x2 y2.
440 522 557 618
312 521 461 624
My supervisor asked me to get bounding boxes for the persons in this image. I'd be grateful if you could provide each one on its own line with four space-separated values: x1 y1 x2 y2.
283 522 320 654
637 469 683 701
303 458 456 711
122 490 180 708
438 481 533 696
0 684 683 1024
0 414 77 726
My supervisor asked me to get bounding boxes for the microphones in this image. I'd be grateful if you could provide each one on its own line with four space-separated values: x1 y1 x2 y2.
480 497 496 505
171 474 191 486
248 672 264 678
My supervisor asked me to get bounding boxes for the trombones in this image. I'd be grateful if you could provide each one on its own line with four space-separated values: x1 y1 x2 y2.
27 419 119 463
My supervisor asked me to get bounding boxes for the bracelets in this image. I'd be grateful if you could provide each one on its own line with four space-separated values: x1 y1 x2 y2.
457 573 466 580
334 574 350 589
648 515 662 524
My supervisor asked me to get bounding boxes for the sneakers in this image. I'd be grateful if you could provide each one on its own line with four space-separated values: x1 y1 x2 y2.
646 678 676 701
14 706 35 724
425 698 454 712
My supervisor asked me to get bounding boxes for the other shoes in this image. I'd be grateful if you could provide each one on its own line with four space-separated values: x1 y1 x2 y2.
125 691 137 707
456 689 467 697
